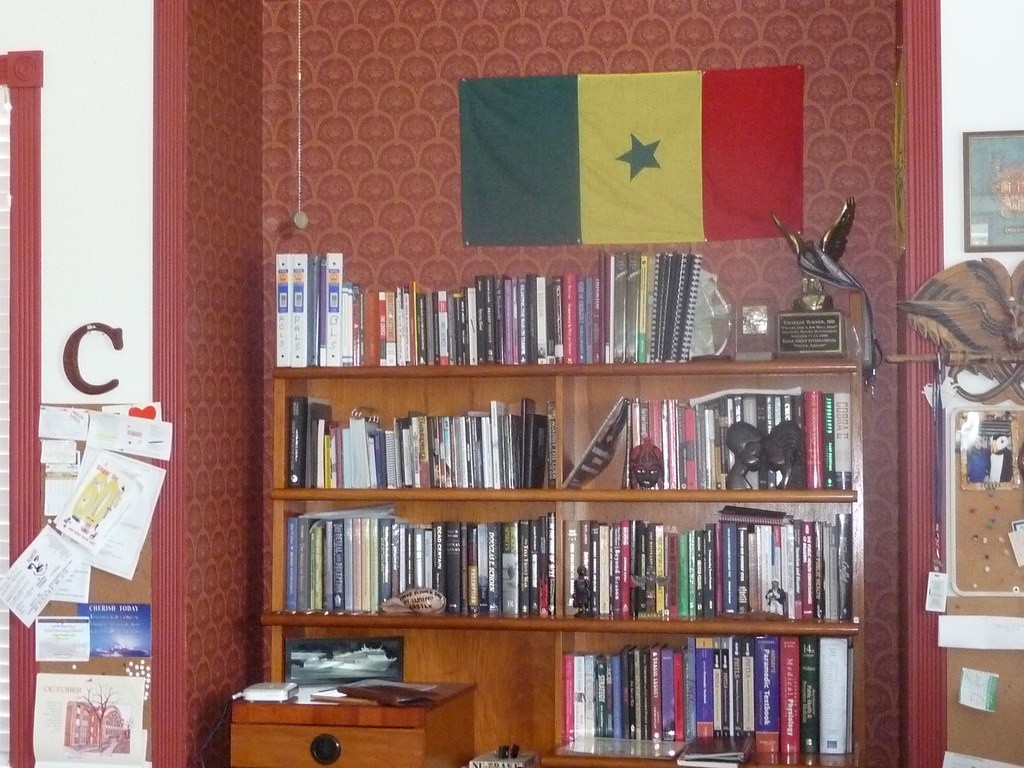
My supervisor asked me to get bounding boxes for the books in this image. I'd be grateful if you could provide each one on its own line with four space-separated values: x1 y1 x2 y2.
243 505 851 767
288 385 850 491
275 250 702 368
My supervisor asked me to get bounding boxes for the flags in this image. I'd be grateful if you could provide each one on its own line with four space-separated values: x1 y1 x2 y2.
457 64 805 246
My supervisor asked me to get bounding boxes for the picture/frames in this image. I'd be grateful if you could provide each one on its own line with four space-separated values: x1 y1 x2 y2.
963 129 1024 253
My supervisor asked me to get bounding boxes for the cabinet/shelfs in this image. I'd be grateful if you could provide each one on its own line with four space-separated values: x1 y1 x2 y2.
229 682 477 768
262 361 862 768
